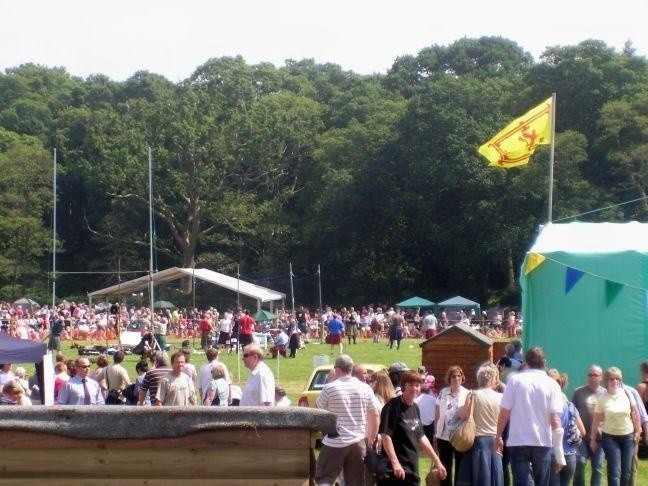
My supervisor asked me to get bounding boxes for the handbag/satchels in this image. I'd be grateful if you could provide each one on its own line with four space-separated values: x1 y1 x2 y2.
565 416 582 447
426 467 440 486
106 387 121 405
364 448 390 482
204 396 219 405
450 414 476 452
121 383 136 402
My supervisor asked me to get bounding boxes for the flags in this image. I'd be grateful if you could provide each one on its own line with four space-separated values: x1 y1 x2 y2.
478 96 553 168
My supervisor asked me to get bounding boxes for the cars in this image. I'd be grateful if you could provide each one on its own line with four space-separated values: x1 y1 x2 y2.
294 363 389 446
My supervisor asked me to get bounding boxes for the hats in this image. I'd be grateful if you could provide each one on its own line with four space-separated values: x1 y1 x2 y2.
388 362 411 374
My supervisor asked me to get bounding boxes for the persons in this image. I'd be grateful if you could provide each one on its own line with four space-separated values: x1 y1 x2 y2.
496 340 523 368
53 342 292 405
1 300 523 359
315 345 648 485
1 363 41 406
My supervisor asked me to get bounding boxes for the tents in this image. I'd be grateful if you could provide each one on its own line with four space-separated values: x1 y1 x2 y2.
395 300 438 319
518 220 648 406
2 332 46 364
438 300 480 318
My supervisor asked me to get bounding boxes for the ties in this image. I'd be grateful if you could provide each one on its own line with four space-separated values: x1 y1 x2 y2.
82 379 91 405
